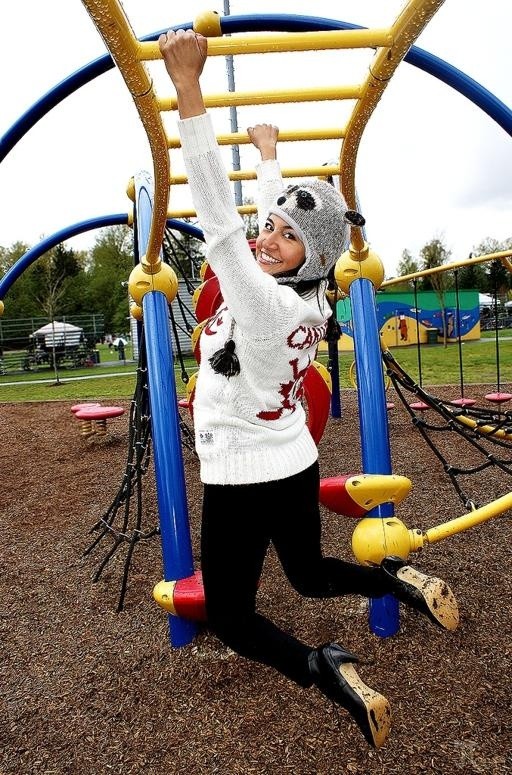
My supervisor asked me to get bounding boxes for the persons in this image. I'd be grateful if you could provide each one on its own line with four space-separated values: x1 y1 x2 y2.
154 22 459 752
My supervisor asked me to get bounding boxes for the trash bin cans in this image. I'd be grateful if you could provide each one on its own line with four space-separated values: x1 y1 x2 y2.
427 330 438 344
90 350 100 363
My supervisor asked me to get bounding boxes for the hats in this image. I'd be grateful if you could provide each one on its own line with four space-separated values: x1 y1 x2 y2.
266 179 366 281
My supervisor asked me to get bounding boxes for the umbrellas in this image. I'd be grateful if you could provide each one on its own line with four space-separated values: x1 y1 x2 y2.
33 320 85 334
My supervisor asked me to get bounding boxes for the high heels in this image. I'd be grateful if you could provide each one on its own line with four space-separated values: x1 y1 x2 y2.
307 642 395 753
380 554 461 634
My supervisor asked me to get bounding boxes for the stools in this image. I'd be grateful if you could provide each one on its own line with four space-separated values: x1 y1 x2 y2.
70 402 124 441
